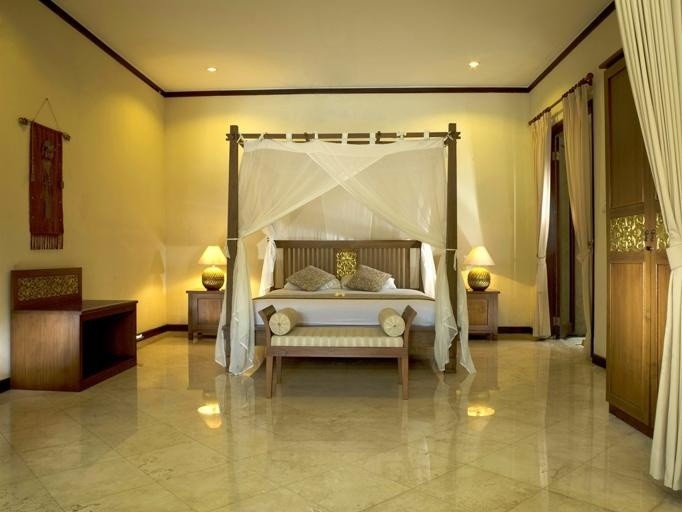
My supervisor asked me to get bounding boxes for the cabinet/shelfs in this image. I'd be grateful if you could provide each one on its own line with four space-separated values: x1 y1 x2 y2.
596 45 671 439
9 264 139 393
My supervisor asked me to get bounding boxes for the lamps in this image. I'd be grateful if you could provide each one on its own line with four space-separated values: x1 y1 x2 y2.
461 245 497 291
196 243 227 290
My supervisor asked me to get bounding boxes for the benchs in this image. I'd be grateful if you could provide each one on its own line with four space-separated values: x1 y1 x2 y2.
258 304 418 400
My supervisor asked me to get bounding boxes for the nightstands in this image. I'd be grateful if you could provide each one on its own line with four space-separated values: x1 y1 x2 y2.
185 290 226 340
465 291 500 341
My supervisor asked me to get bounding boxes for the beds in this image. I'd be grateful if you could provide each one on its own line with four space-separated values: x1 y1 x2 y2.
222 123 461 374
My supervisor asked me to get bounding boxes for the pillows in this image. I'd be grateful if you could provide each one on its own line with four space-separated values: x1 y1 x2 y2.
345 264 392 293
284 264 334 292
285 279 341 291
340 278 397 291
